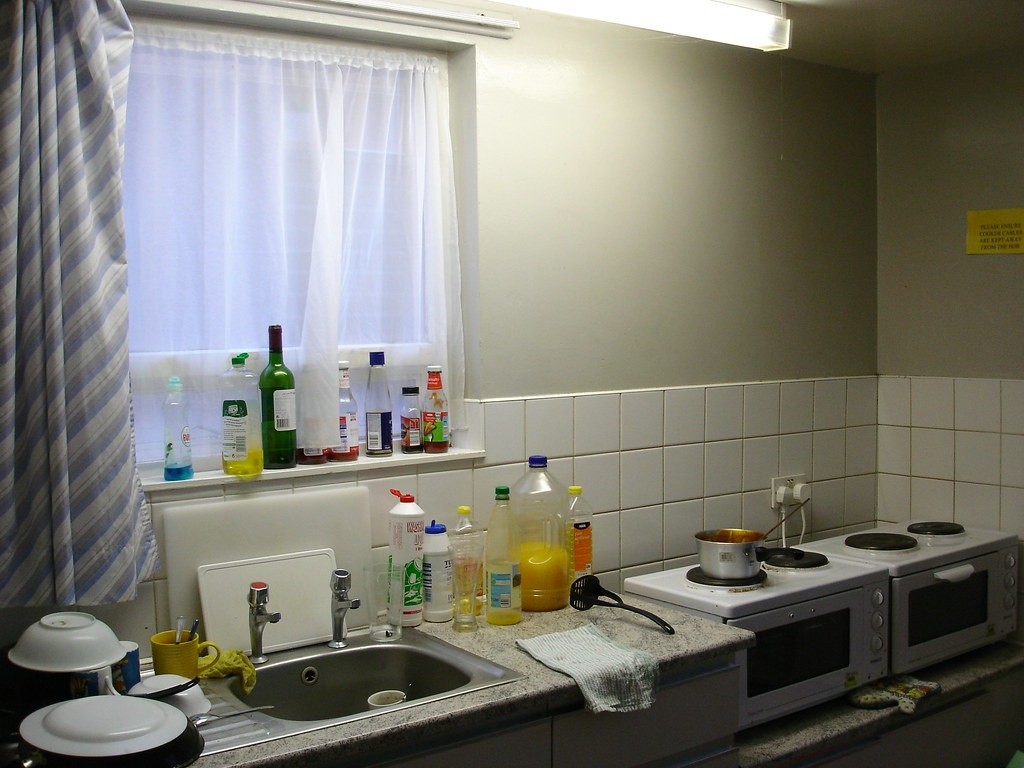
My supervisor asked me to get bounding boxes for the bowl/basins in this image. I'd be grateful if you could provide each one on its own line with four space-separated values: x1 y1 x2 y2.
125 672 214 719
6 611 128 673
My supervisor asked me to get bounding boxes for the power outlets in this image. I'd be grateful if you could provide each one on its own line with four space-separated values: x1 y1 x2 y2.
770 469 814 510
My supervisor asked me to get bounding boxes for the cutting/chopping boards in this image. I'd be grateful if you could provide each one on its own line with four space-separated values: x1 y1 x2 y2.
164 486 374 651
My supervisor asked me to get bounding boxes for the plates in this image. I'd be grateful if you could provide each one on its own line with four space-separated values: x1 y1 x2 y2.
18 694 187 756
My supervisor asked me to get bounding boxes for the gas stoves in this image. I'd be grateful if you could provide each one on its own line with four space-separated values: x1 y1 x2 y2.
621 544 888 618
791 520 1018 578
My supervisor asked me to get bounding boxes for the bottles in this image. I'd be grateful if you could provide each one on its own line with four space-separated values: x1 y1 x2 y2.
296 360 360 464
220 353 264 476
484 486 522 625
564 486 593 586
508 454 570 612
422 365 450 454
259 325 296 469
388 487 425 627
364 352 394 457
162 376 194 481
452 506 482 615
423 520 453 623
400 386 423 454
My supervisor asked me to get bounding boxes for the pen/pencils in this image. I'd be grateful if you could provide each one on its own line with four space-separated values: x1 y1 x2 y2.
175 616 186 642
188 620 200 641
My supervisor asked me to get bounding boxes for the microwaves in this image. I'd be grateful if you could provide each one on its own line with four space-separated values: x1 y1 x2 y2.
623 570 888 732
891 545 1018 678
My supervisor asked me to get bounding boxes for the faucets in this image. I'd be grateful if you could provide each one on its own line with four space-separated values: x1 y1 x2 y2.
328 569 360 649
246 581 282 664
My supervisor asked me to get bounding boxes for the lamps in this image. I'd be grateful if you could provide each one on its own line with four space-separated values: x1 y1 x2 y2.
504 0 795 61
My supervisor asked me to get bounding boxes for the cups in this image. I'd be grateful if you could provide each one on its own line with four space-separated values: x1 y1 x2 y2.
111 640 141 696
367 690 407 711
361 562 404 642
151 630 222 680
448 541 484 632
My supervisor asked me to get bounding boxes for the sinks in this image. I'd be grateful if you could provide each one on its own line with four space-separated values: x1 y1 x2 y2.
228 644 474 720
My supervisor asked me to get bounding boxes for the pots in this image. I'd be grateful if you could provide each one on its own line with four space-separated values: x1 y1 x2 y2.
5 677 206 768
694 528 804 578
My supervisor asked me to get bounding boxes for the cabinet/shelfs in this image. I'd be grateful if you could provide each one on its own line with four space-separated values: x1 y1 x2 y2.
383 667 739 768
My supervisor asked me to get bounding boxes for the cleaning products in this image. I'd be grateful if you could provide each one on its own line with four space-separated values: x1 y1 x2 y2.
220 352 263 475
162 377 195 482
388 488 426 629
